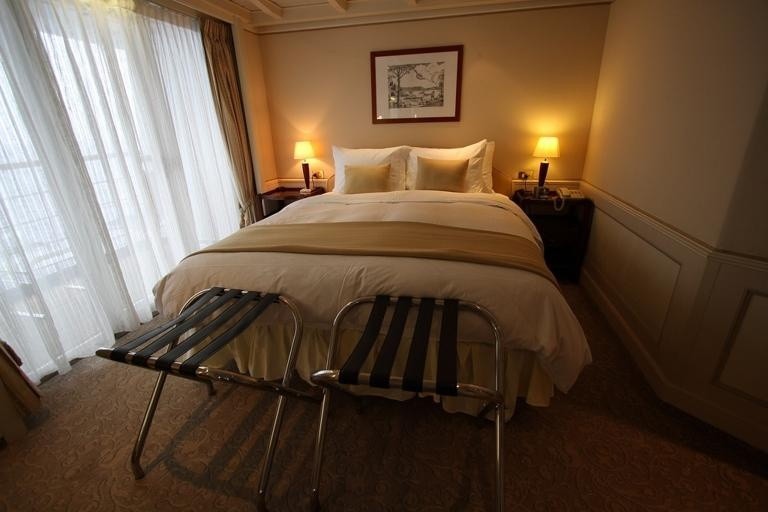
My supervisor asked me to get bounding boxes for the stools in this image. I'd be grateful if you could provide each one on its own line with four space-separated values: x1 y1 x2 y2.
96 288 315 512
310 294 507 512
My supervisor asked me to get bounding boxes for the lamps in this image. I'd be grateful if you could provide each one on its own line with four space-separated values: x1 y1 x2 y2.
293 141 316 195
533 136 560 194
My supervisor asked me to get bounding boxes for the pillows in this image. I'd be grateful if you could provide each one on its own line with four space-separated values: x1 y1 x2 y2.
331 139 496 193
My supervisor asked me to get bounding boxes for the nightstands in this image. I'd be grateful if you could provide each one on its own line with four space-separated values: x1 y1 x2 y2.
511 189 595 287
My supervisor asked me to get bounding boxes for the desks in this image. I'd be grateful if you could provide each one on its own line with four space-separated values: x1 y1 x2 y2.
261 186 323 201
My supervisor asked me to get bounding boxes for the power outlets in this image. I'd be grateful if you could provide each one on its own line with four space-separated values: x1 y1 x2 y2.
520 172 526 180
317 171 324 179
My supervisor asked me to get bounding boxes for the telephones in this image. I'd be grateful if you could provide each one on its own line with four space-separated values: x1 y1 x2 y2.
557 187 585 200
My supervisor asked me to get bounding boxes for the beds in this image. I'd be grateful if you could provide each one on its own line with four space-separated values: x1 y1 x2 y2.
157 167 594 423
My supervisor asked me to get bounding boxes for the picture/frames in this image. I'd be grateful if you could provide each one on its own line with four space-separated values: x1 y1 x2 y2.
371 45 464 124
